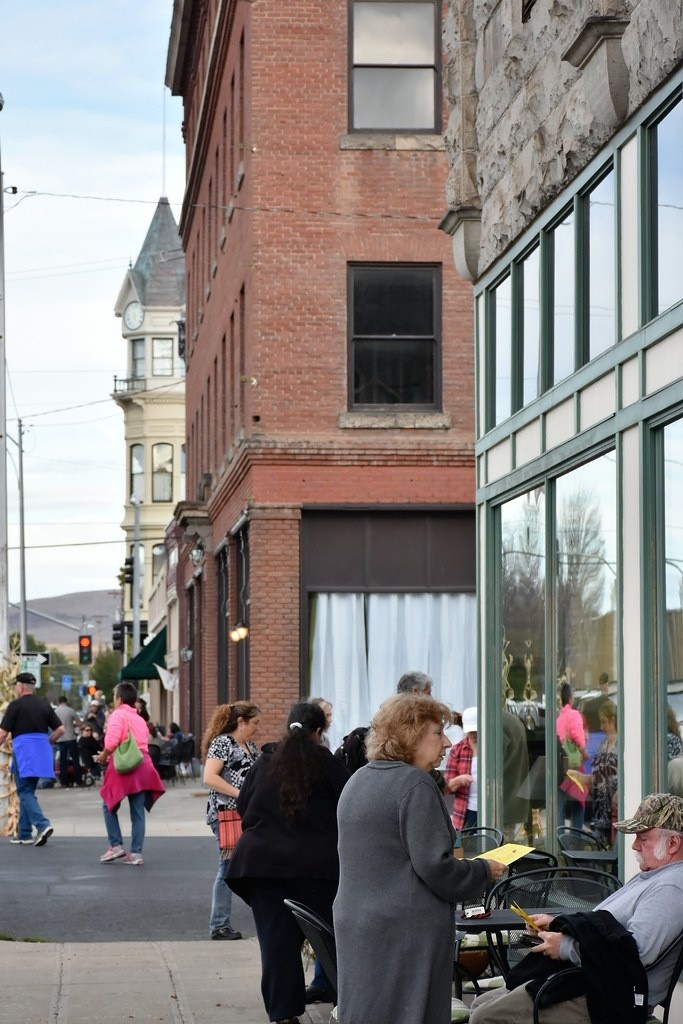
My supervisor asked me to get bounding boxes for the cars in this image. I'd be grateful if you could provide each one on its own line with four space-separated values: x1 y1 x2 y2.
560 676 683 762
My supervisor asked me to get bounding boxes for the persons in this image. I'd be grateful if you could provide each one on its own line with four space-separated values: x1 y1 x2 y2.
502 663 683 852
222 702 351 1024
0 673 65 847
202 671 477 1001
38 690 194 788
469 793 683 1024
332 692 509 1024
96 683 166 865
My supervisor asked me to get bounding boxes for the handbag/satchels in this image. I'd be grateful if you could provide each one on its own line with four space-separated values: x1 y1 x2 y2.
113 733 143 774
561 741 582 771
217 809 244 850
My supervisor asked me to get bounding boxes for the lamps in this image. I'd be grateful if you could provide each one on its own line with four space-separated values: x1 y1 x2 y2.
182 646 194 663
188 542 205 568
229 619 250 643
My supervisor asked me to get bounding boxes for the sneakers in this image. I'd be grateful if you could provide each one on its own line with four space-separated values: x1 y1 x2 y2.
303 984 334 1004
32 823 54 846
211 926 242 941
9 833 34 845
99 844 126 862
121 852 145 865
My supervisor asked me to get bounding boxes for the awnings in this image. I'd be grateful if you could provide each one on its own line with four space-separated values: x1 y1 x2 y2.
117 632 166 682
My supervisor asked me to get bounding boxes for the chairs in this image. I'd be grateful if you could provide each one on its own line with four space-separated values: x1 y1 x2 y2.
280 826 683 1024
84 738 196 787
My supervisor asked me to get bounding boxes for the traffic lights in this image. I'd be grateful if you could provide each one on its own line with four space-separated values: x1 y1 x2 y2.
112 622 124 653
88 686 99 695
124 556 134 584
80 686 87 696
78 635 93 665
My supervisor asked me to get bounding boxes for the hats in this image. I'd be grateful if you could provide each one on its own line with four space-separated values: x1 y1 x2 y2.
58 696 68 703
8 673 36 685
461 706 478 733
610 794 683 834
90 700 99 706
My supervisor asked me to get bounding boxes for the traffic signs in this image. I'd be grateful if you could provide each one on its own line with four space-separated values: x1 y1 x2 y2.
12 652 52 667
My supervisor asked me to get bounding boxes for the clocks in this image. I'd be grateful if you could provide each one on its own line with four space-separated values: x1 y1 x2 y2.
123 301 146 328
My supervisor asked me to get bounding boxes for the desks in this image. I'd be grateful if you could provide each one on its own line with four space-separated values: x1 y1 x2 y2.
450 909 608 1000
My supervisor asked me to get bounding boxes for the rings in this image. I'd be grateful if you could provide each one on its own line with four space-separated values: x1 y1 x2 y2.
543 951 547 956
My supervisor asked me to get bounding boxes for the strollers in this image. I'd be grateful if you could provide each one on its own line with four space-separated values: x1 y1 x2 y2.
55 748 94 786
